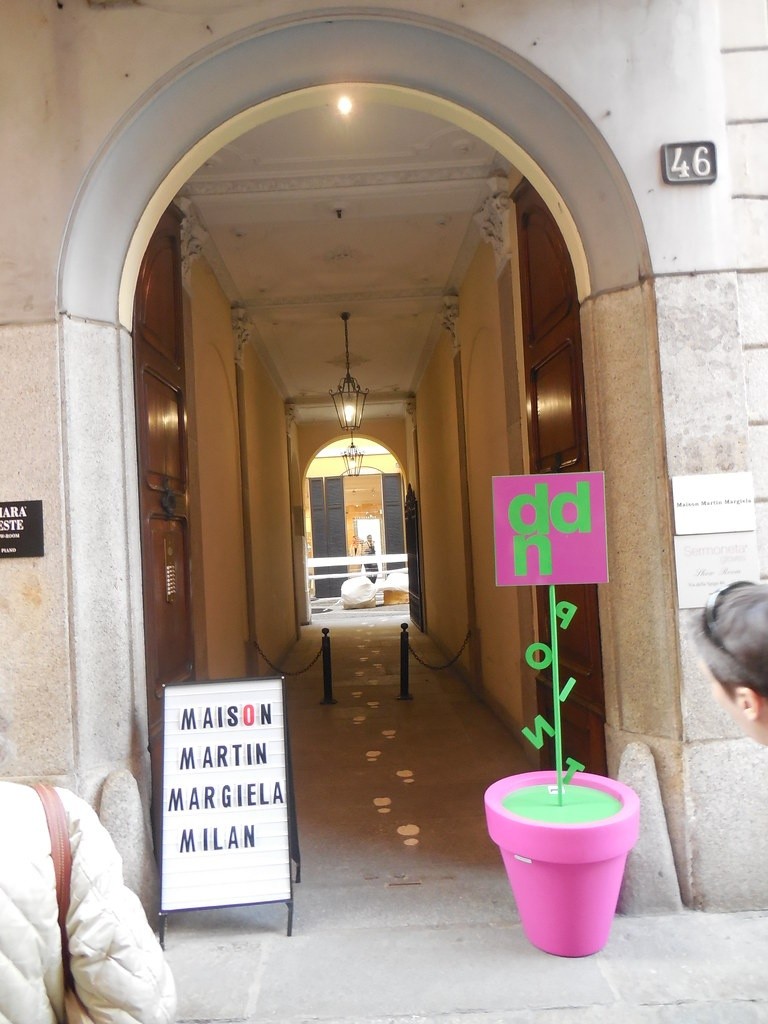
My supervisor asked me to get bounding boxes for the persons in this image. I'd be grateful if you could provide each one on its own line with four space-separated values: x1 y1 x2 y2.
0 780 177 1024
364 534 379 584
676 580 768 747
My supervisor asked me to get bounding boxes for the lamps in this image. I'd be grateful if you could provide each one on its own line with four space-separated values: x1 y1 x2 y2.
327 312 370 431
339 429 364 477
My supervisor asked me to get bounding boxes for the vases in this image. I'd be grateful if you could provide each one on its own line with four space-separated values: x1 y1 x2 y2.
482 771 642 957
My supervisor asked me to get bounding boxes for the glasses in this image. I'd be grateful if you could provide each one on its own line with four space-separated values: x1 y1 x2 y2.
703 582 756 669
